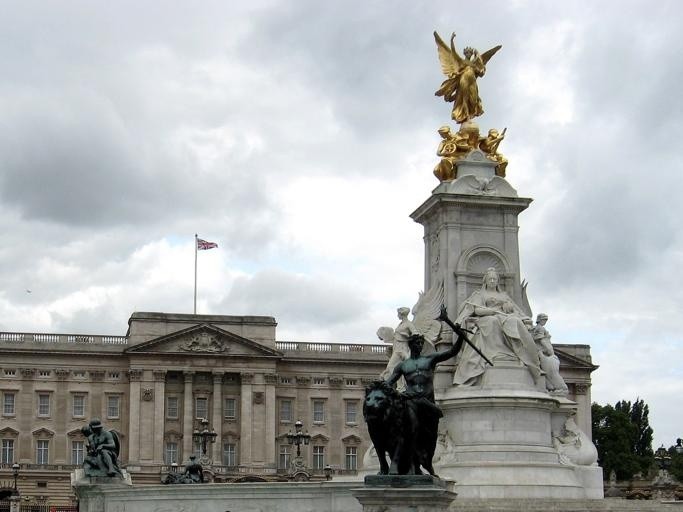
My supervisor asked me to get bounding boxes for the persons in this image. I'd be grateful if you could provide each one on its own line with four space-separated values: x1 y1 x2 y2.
450 31 486 124
534 312 565 390
457 266 542 385
387 325 466 472
481 129 508 178
85 418 118 476
433 125 461 180
182 454 204 482
380 307 413 384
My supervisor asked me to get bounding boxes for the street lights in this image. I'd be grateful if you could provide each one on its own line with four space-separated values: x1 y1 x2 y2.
323 465 331 481
10 462 20 496
287 419 311 456
192 417 217 455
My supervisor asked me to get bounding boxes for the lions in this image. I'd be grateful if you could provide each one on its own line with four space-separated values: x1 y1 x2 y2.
362 378 438 476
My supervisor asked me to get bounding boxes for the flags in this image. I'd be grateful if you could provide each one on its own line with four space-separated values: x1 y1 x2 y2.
198 239 218 250
